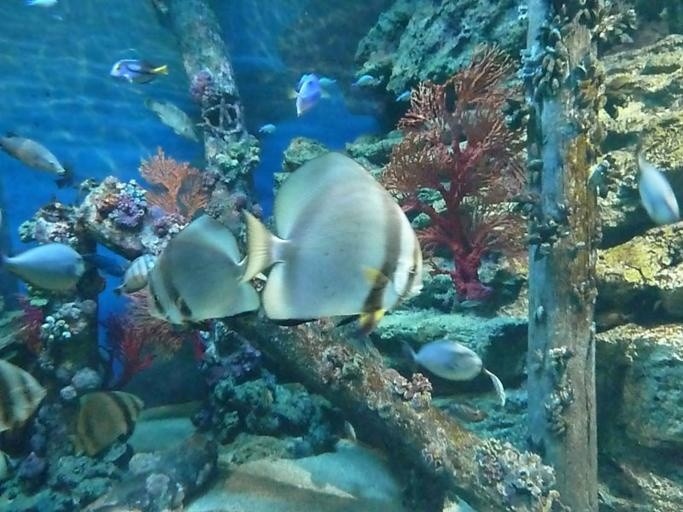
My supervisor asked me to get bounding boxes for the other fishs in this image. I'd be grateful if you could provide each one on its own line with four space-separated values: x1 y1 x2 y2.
0 359 51 484
